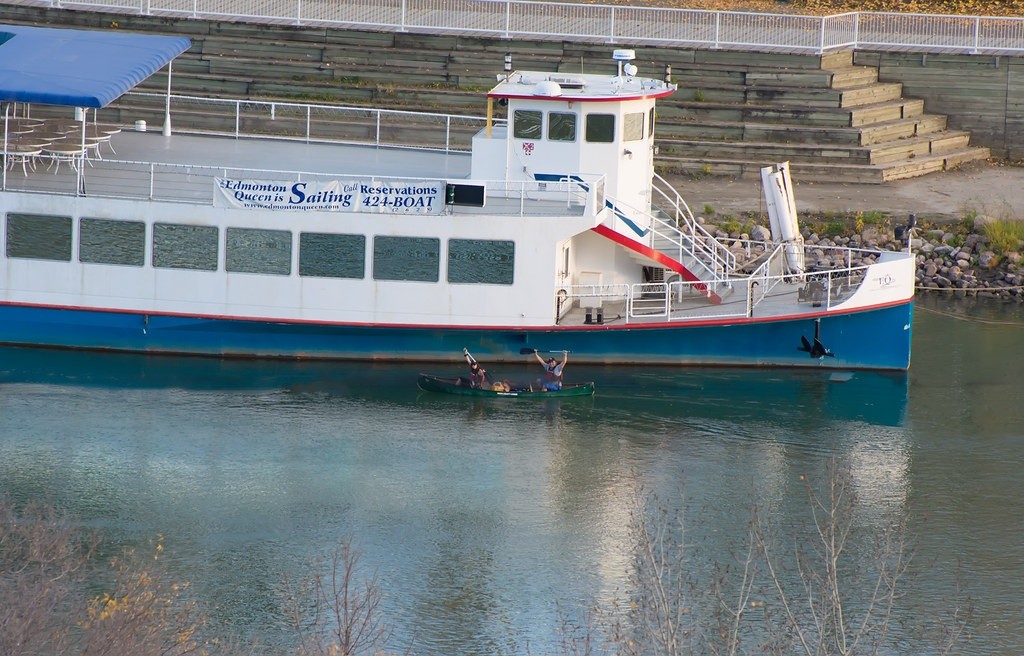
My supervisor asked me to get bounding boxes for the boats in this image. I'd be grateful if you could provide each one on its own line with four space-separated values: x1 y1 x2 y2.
0 20 917 372
418 374 597 397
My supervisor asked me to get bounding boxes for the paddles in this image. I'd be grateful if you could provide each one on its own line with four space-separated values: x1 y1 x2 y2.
518 348 574 355
464 349 492 382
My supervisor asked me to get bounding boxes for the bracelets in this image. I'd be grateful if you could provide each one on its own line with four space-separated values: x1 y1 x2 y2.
463 353 469 357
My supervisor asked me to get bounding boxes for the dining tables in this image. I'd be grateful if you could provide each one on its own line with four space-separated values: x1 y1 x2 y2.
1 116 121 174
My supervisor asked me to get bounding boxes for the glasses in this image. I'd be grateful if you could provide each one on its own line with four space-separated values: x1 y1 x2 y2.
471 363 477 366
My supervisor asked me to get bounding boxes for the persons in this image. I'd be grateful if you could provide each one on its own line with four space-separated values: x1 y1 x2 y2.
456 348 485 388
211 176 446 217
534 349 568 392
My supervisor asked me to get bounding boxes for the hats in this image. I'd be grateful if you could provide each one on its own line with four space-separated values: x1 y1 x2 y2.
547 357 555 363
